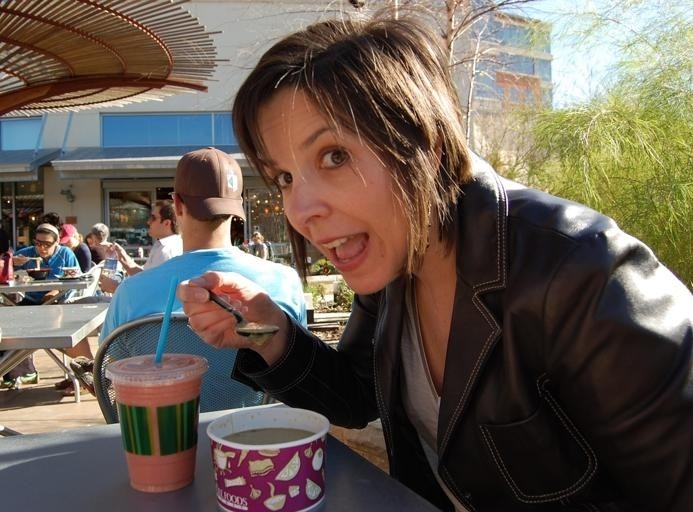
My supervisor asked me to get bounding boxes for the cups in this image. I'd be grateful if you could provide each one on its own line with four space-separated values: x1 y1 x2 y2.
102 354 208 495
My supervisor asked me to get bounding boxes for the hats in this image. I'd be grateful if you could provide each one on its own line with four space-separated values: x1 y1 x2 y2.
60 224 79 243
173 147 248 221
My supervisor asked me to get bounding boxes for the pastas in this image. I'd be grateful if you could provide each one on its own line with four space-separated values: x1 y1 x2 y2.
35 257 44 271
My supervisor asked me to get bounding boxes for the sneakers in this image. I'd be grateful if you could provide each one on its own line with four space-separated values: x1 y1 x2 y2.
55 354 101 397
0 373 41 393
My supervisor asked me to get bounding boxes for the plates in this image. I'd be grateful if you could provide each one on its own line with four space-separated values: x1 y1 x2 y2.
54 275 80 281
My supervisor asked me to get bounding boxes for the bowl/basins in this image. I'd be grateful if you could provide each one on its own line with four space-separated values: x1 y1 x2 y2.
60 267 80 277
204 407 330 509
26 268 50 279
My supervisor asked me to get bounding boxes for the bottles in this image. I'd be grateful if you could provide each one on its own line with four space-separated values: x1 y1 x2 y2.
100 243 118 279
137 246 144 257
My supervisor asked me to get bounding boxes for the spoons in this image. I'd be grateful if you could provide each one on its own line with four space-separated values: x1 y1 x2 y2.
206 286 282 338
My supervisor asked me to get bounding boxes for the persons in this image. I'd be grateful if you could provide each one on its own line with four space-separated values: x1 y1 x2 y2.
174 4 693 512
98 147 308 416
1 222 84 388
252 231 268 259
1 212 119 297
54 203 183 395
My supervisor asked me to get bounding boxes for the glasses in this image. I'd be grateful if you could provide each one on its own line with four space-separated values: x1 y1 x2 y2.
146 213 163 224
34 239 58 248
